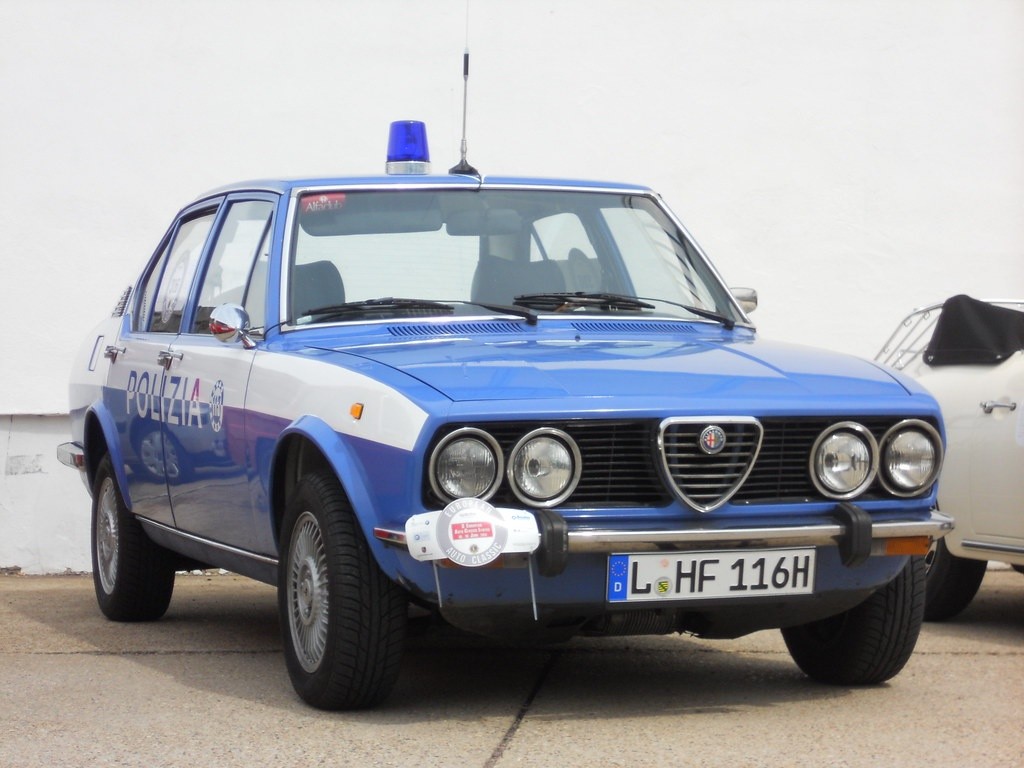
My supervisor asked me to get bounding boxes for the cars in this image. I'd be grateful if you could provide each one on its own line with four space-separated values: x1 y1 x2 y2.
54 121 958 713
872 296 1024 620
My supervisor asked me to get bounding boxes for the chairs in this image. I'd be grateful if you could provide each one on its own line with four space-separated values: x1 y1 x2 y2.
291 260 345 321
470 255 566 309
